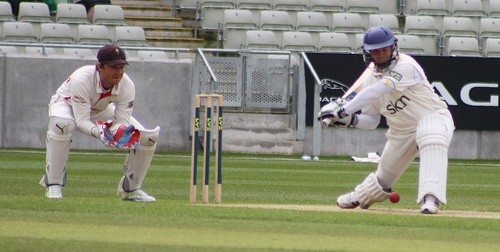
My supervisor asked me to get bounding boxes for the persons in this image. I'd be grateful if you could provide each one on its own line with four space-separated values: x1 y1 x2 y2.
316 26 454 213
40 45 160 202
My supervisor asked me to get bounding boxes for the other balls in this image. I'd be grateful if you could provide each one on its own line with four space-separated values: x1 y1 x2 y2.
390 192 400 202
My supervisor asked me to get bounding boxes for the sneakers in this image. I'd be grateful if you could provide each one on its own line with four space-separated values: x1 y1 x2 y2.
44 183 64 199
336 192 361 209
419 195 442 214
118 189 157 202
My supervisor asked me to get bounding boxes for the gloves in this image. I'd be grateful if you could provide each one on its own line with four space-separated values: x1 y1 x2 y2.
316 98 356 129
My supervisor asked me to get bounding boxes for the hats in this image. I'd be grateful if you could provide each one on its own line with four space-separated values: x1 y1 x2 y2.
97 44 129 67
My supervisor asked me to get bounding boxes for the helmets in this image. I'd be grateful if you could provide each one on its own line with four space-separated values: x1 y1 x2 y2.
361 25 398 51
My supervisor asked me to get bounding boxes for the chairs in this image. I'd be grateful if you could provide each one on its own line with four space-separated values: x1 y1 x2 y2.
0 0 147 53
173 0 500 57
